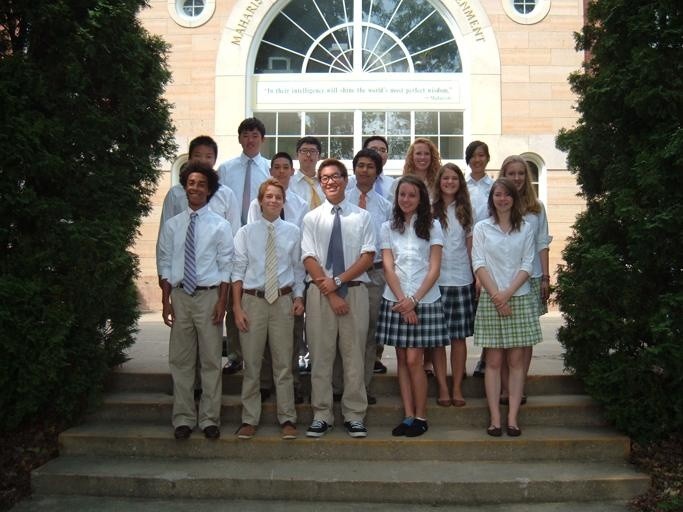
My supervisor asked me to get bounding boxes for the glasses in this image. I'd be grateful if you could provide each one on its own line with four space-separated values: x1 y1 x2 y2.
298 149 318 155
321 174 340 183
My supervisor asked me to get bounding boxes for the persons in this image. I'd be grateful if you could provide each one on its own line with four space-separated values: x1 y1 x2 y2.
161 118 329 402
231 179 307 438
156 162 233 439
429 163 482 406
375 175 450 437
332 136 396 404
472 178 544 434
499 156 552 406
463 139 494 376
301 159 377 438
390 137 443 376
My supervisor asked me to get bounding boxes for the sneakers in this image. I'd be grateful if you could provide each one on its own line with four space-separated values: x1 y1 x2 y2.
345 421 368 438
295 391 304 402
368 394 377 404
280 423 297 439
306 420 333 437
260 388 270 401
374 361 387 374
238 424 255 438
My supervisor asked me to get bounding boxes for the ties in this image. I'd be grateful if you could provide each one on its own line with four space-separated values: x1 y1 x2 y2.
241 159 251 224
325 207 348 299
359 191 366 209
264 224 279 305
182 213 197 295
304 176 321 209
374 177 382 195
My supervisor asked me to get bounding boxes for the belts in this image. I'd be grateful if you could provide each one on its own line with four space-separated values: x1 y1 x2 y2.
179 283 217 291
368 262 383 270
348 281 360 287
244 287 291 298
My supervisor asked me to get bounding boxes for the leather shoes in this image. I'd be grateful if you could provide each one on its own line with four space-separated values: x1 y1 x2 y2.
500 394 527 405
508 426 521 436
438 398 465 407
472 361 485 376
174 426 191 439
222 360 243 372
205 425 219 438
487 424 501 436
392 418 428 437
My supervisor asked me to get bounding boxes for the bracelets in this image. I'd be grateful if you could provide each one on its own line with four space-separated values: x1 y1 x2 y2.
543 275 550 280
411 295 420 306
